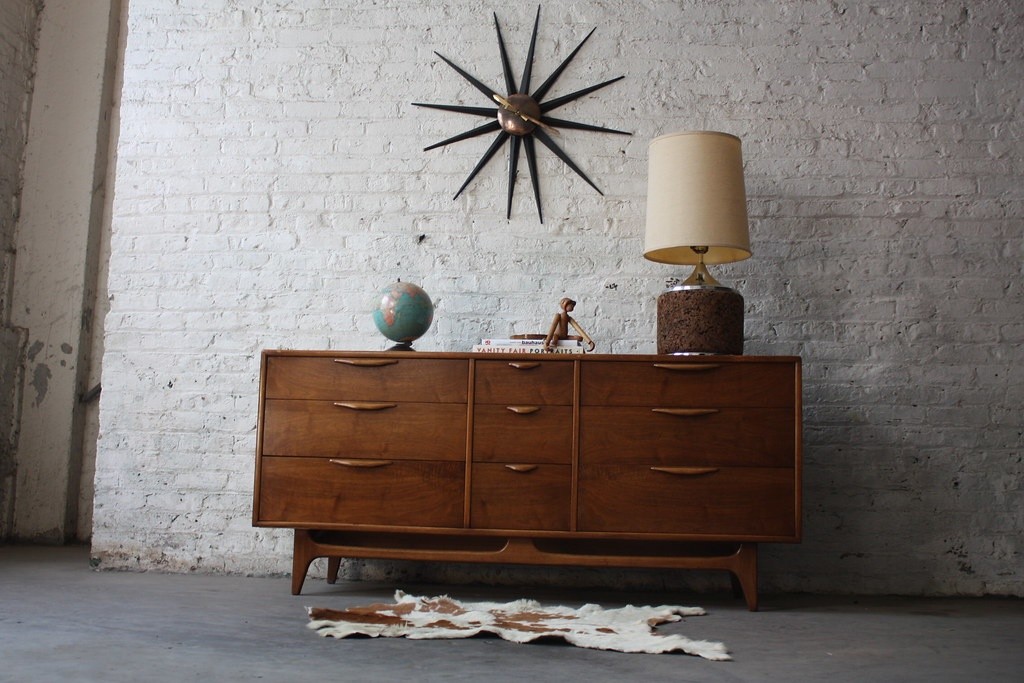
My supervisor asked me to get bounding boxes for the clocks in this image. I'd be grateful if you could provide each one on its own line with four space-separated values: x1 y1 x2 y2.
411 2 631 225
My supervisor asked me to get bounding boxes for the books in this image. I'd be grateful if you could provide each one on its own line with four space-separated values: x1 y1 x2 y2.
471 336 585 355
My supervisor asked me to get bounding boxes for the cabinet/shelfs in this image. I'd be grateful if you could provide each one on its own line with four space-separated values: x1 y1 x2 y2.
252 349 800 613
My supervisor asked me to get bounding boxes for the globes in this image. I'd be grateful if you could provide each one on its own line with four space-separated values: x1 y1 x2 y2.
372 278 433 352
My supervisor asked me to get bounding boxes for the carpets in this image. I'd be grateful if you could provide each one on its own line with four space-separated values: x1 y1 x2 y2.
305 589 733 662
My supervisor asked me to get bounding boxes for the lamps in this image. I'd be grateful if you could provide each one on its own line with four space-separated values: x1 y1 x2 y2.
644 129 755 358
